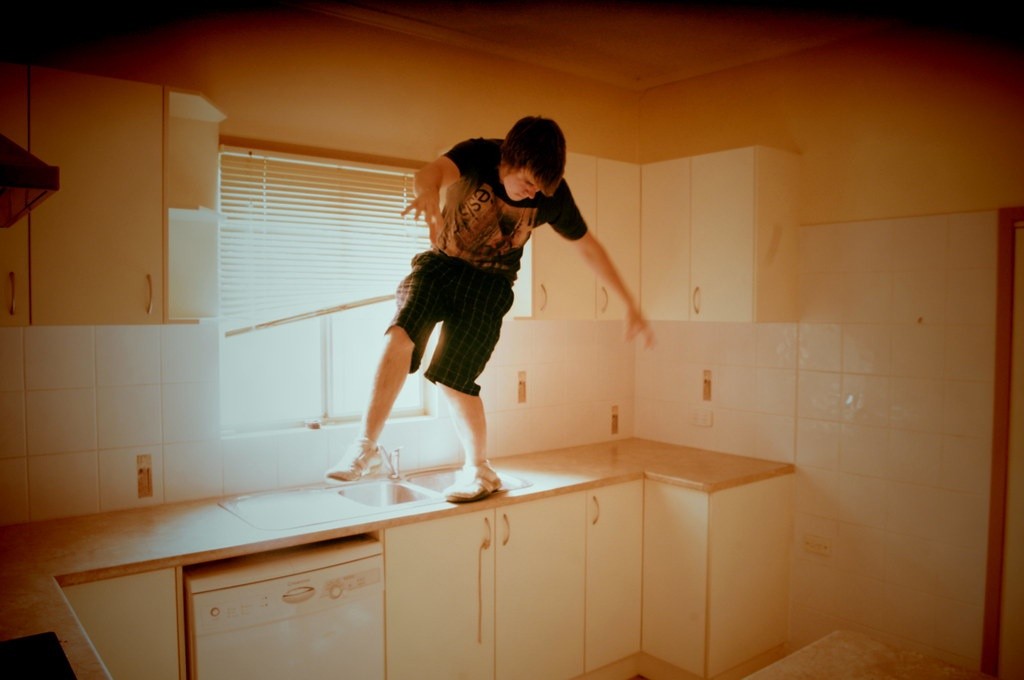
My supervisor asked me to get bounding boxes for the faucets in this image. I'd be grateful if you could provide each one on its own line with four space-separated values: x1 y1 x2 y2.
379 445 399 479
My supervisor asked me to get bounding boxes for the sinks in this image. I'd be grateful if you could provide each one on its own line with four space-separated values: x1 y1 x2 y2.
403 467 534 499
324 480 432 512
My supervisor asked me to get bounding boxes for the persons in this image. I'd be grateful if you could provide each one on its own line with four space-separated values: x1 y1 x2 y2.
325 115 656 503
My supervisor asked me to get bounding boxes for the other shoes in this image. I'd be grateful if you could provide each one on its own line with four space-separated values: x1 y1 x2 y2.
328 437 380 482
442 460 503 503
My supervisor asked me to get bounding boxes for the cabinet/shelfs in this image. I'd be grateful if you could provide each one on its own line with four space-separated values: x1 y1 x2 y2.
503 152 642 322
0 63 226 326
641 146 807 323
380 478 792 680
63 565 186 679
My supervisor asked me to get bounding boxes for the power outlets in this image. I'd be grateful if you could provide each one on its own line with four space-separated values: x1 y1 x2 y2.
803 533 831 556
